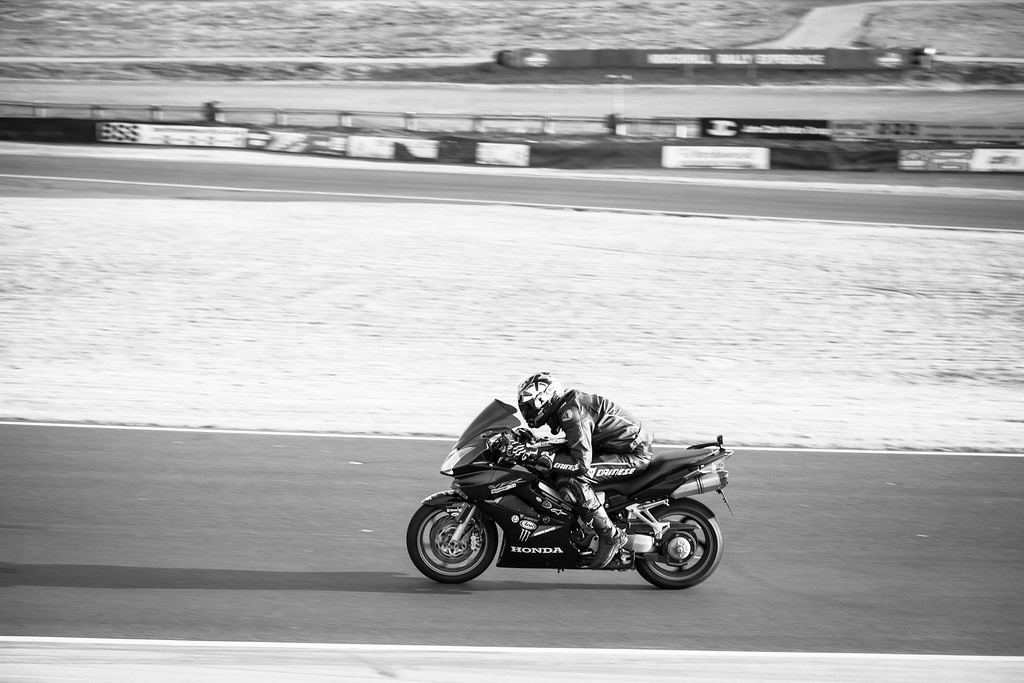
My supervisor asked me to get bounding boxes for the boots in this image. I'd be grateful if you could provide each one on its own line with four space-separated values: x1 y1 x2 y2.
584 504 628 571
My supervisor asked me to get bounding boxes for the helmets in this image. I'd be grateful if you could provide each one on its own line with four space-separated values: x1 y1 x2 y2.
517 372 561 428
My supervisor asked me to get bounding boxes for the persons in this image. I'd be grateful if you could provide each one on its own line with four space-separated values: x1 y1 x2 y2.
507 372 655 568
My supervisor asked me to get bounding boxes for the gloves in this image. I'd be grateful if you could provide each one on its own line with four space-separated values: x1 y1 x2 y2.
515 426 534 442
510 442 540 466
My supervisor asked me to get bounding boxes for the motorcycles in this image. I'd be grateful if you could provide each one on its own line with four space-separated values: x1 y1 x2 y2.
406 399 736 590
